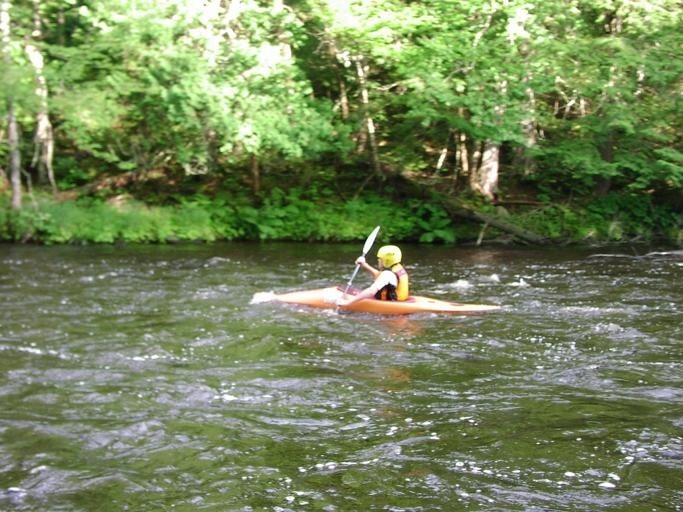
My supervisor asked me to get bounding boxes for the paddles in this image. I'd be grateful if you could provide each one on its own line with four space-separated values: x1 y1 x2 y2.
344 226 381 293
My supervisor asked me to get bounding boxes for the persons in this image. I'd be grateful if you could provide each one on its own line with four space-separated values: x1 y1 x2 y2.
336 244 409 306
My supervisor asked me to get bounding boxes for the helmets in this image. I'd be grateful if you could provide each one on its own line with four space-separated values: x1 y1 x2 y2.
376 245 403 269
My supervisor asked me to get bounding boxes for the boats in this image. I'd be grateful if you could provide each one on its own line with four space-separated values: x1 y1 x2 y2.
276 285 502 316
